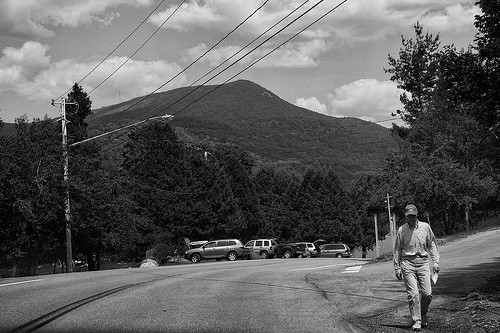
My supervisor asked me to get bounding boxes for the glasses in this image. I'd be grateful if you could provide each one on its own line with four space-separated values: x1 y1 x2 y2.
408 215 416 216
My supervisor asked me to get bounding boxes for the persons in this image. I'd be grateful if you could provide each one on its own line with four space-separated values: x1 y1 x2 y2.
393 204 440 330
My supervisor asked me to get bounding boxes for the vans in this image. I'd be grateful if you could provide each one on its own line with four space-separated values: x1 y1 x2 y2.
245 239 281 260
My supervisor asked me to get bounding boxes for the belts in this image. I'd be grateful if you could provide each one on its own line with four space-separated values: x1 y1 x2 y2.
407 255 426 257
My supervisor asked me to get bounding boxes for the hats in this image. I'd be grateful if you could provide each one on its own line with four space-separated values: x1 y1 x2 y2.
404 204 418 217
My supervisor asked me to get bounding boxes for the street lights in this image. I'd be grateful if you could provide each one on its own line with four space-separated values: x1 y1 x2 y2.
62 113 175 270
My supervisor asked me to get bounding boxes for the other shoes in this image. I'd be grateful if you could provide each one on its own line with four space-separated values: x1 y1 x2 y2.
422 319 428 326
412 321 422 330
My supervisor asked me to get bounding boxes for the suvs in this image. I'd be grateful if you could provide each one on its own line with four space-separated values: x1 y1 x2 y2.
184 239 245 263
278 242 353 259
